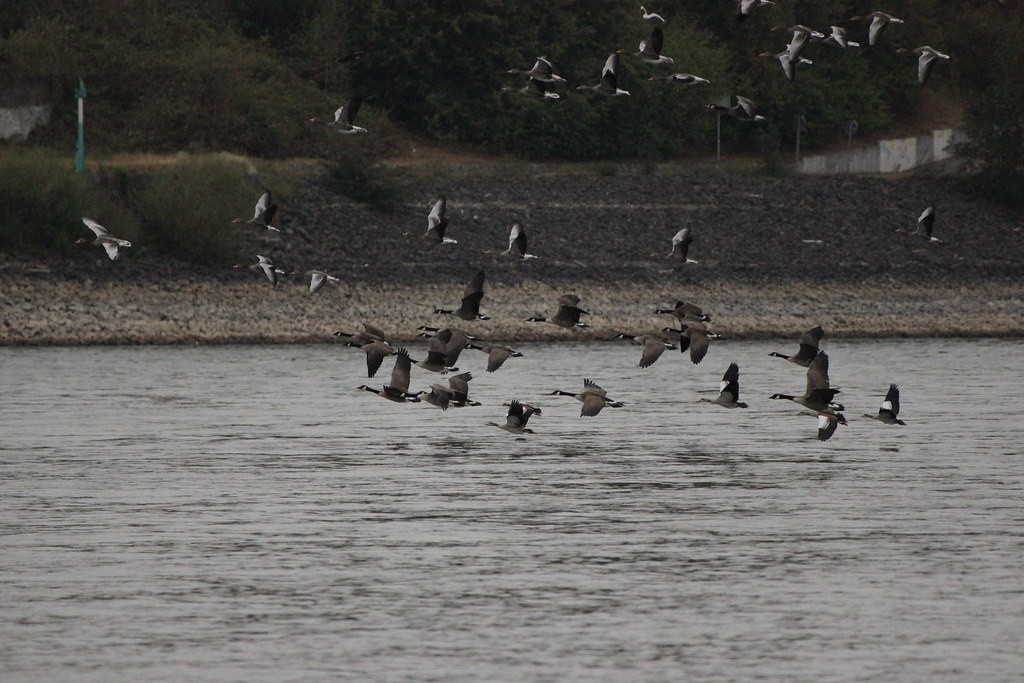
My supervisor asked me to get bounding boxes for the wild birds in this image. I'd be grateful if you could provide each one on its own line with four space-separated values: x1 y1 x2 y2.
742 1 951 85
614 224 749 409
501 7 767 124
768 326 905 441
332 196 624 436
894 206 943 246
75 99 367 292
